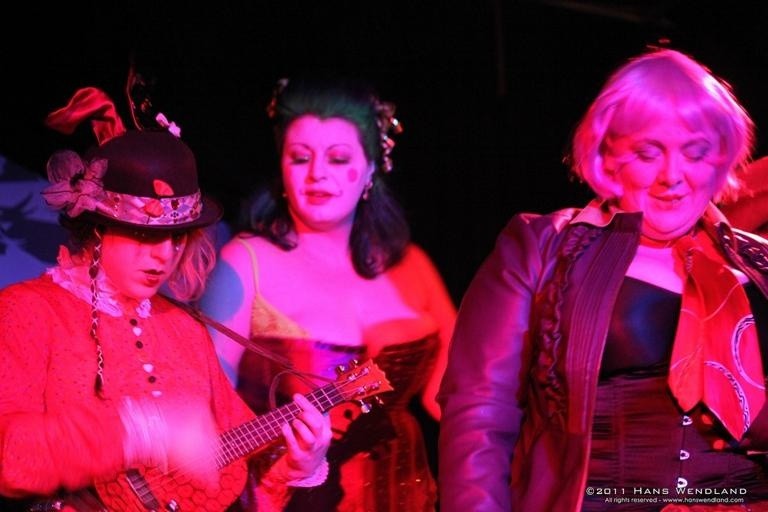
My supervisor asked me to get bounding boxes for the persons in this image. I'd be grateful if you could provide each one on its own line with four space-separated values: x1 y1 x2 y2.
435 47 768 512
197 87 457 512
2 128 333 512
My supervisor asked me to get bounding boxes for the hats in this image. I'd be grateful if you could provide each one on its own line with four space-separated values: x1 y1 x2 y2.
62 130 225 231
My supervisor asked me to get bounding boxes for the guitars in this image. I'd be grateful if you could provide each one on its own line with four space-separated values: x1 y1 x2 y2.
94 359 394 511
